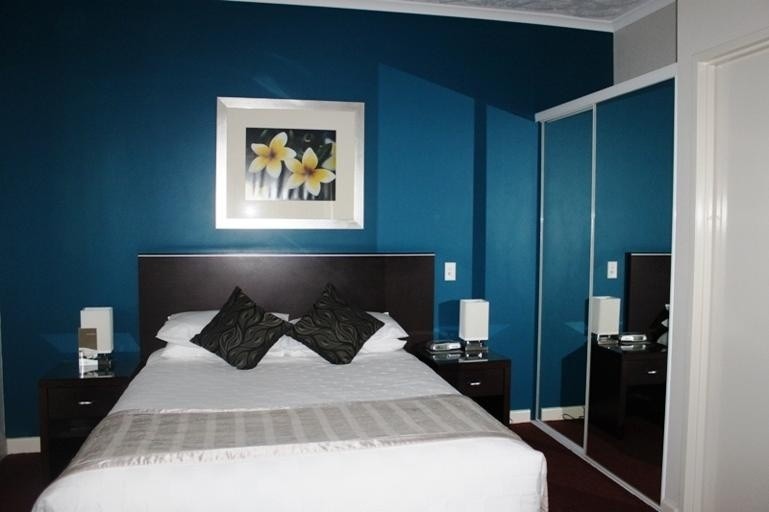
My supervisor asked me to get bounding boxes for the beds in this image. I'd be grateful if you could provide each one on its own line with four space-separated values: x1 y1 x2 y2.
28 252 547 511
627 253 671 342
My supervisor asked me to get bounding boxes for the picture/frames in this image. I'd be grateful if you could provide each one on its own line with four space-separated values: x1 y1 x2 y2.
214 96 365 230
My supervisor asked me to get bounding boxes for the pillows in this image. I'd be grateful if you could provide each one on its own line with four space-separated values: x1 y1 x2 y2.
656 303 669 347
153 284 410 369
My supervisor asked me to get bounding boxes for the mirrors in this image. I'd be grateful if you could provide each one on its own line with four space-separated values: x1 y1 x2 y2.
536 64 675 511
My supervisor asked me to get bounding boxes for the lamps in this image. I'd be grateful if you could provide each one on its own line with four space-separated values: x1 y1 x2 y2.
78 307 114 372
459 297 490 356
592 296 621 344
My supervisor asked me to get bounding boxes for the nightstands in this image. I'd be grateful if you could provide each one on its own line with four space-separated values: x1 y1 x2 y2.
590 337 664 437
40 361 140 484
419 346 512 427
